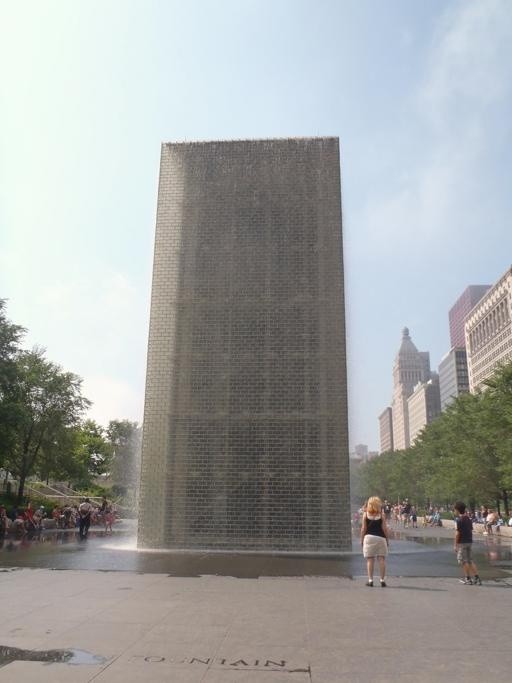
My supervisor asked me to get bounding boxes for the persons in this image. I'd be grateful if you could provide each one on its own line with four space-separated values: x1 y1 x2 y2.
0 494 139 538
453 502 481 586
351 497 512 536
360 494 389 587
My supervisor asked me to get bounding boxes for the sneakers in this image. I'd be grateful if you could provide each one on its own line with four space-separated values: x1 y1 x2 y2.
456 575 472 585
366 581 373 586
471 574 481 585
380 581 386 587
